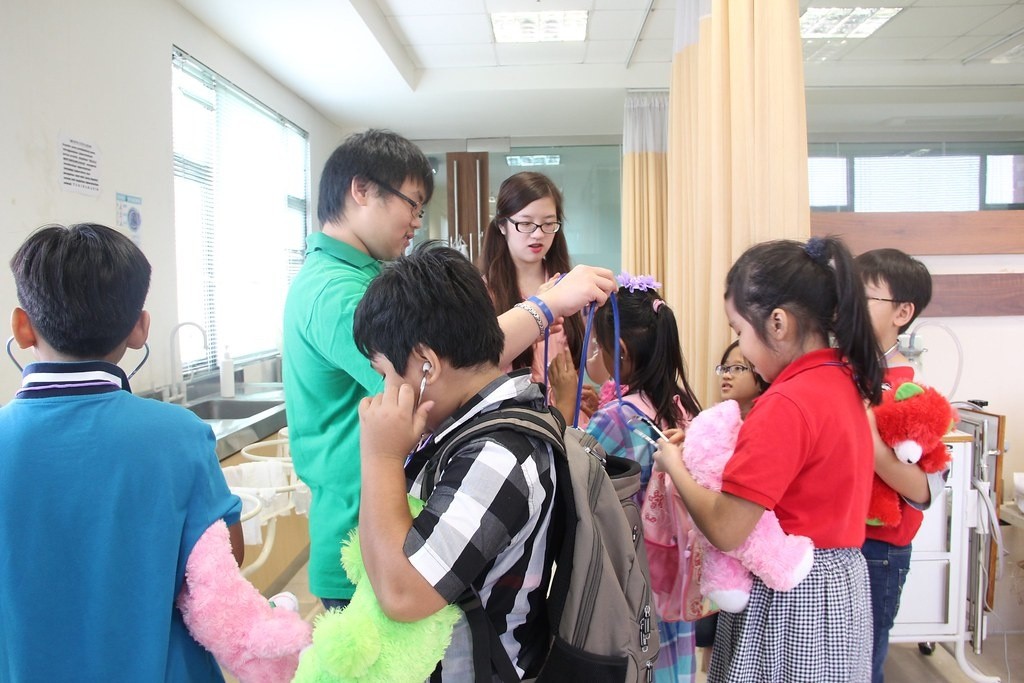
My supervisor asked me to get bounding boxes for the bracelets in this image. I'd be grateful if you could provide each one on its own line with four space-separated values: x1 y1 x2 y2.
526 295 554 325
514 302 545 337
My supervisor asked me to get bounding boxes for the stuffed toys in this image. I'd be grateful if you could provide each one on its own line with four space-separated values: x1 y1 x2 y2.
862 384 961 530
175 518 313 683
314 495 471 624
683 399 816 616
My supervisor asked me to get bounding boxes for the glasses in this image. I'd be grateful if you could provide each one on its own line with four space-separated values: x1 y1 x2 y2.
360 171 424 220
865 295 899 303
505 218 563 235
714 363 749 376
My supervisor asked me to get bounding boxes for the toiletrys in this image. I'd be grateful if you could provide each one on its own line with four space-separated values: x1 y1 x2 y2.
219 343 235 398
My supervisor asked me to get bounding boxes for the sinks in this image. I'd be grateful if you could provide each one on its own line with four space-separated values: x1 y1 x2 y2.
186 400 285 420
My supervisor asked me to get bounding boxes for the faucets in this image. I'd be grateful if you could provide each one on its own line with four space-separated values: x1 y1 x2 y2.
151 321 209 408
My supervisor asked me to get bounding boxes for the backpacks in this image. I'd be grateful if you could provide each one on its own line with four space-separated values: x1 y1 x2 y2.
607 391 721 623
422 403 661 683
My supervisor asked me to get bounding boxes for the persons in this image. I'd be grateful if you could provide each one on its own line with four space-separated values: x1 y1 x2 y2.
1 222 251 644
653 237 949 682
282 129 701 682
